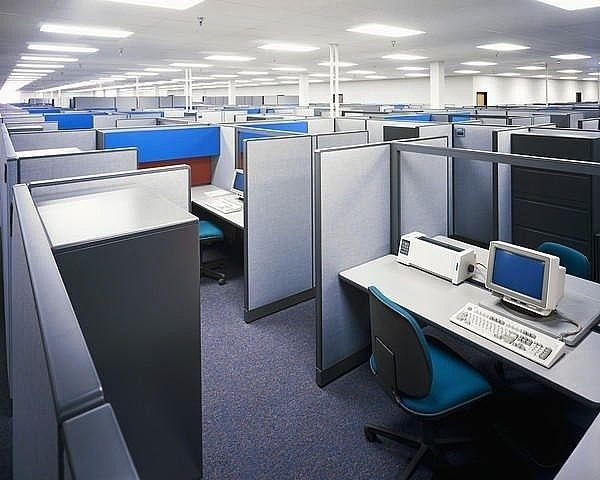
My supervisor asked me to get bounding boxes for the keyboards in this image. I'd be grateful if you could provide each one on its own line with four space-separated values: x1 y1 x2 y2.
204 197 242 214
448 301 566 369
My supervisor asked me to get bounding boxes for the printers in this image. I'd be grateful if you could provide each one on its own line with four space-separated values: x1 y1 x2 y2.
395 230 477 286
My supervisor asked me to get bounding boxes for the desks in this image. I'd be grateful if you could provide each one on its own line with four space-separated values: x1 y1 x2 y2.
189 184 244 232
338 235 600 410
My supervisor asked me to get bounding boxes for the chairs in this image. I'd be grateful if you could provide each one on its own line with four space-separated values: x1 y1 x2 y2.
361 285 497 480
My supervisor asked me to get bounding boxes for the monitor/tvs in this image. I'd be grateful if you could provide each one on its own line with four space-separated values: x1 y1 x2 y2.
485 240 566 321
229 168 244 200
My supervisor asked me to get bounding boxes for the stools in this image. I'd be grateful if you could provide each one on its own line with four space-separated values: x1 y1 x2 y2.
199 218 227 285
